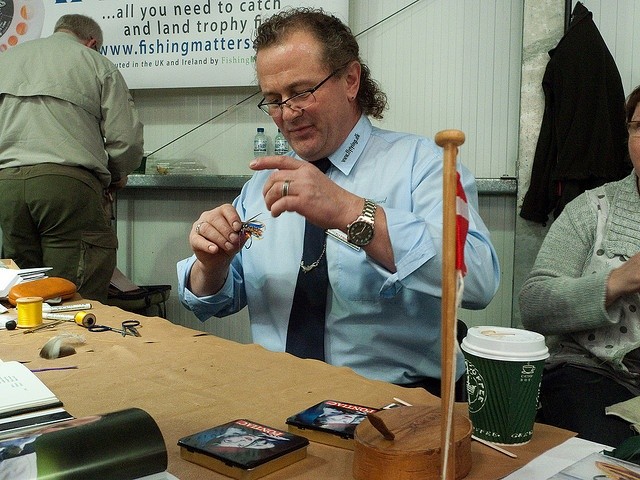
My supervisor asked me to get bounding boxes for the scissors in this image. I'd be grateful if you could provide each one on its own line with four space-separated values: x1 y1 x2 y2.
89 320 140 337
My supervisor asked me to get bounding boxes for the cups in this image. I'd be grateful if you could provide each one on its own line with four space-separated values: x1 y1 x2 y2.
459 325 551 448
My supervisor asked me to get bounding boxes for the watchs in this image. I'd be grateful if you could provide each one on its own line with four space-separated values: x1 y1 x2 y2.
346 198 377 247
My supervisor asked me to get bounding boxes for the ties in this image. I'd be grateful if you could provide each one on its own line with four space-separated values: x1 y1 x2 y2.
285 157 332 363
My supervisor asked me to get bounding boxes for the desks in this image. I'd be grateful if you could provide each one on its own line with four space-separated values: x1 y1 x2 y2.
0 264 638 480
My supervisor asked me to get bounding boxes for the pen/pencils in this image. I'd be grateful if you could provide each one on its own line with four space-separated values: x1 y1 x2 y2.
42 303 92 312
41 311 75 322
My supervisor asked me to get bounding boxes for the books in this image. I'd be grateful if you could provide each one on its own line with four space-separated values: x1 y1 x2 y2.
0 360 77 438
605 396 640 434
0 407 184 479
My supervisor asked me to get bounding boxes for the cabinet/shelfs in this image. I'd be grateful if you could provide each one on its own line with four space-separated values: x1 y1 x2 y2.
114 174 515 194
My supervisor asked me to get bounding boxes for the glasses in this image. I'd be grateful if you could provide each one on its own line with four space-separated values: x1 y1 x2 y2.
257 64 349 117
625 118 640 137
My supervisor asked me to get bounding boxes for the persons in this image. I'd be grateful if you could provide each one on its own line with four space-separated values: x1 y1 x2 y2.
0 14 143 305
176 5 501 403
518 84 640 447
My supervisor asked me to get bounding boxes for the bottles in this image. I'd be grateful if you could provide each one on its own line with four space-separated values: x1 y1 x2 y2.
274 129 290 156
253 127 267 159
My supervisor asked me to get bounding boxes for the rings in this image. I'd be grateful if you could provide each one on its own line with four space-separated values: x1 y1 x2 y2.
282 180 290 197
195 221 206 234
